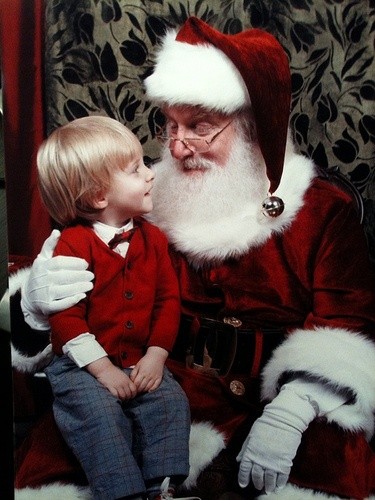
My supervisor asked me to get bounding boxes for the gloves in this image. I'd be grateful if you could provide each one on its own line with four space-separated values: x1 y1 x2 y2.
17 229 96 331
236 390 317 494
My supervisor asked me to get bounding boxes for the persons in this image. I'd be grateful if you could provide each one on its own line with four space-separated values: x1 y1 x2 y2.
0 16 375 500
37 116 192 500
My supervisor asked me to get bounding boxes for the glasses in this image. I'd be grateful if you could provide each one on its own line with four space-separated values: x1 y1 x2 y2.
154 111 239 152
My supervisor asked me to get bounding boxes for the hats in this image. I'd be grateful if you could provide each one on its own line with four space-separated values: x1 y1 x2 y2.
143 15 293 219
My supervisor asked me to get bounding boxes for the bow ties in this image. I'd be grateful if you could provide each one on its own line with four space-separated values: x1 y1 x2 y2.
107 228 136 250
